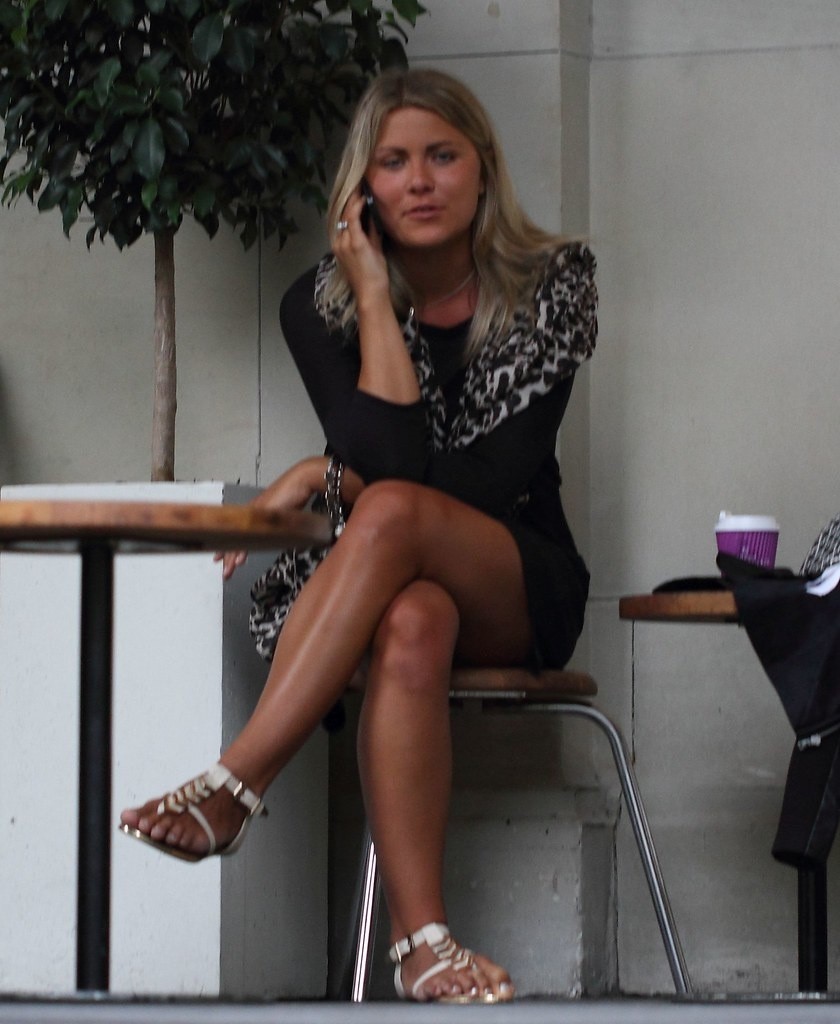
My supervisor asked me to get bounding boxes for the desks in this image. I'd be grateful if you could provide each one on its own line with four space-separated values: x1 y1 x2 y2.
619 588 840 1002
0 498 333 1006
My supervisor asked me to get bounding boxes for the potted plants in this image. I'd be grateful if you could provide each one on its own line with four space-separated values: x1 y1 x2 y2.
0 0 429 1004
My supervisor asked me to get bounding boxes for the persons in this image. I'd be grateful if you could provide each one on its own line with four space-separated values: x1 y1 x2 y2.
116 66 602 1004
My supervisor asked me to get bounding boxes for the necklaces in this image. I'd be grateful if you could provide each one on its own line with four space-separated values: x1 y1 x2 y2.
407 270 474 316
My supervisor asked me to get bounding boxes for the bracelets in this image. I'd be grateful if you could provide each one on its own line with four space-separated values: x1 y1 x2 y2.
324 458 350 523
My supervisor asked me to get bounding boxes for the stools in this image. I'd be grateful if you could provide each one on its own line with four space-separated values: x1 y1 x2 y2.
336 664 692 1002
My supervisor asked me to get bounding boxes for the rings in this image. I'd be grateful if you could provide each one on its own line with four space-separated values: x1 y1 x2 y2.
338 222 349 229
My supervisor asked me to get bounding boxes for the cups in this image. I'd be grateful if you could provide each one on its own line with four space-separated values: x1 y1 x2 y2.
714 513 779 568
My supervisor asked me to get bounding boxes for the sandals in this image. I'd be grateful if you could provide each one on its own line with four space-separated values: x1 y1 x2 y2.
119 762 269 862
389 922 516 1005
